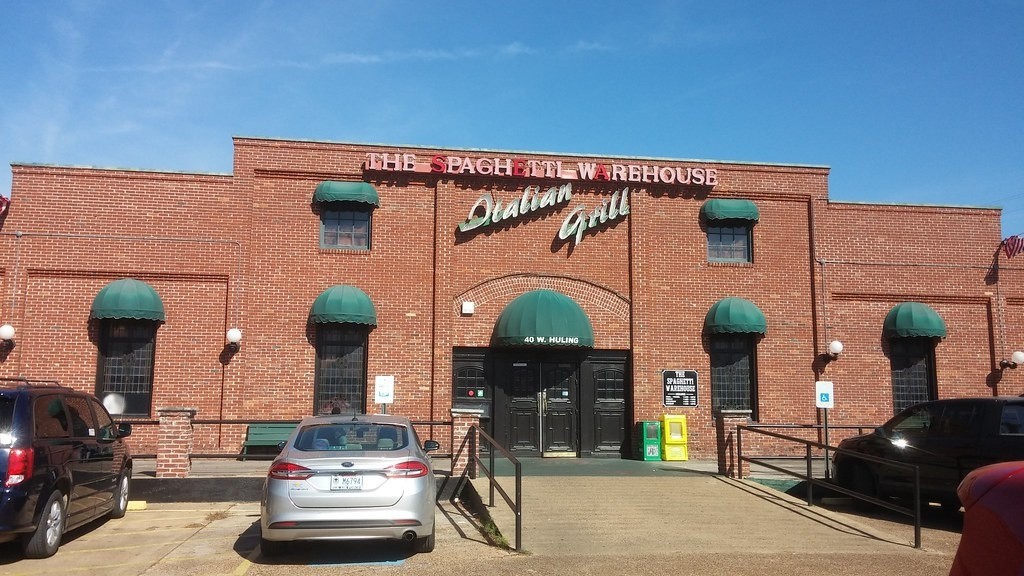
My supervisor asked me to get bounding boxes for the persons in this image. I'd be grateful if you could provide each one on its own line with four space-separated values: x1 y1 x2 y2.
317 407 345 445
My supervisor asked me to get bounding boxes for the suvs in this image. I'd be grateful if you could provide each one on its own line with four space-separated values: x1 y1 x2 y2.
0 377 133 562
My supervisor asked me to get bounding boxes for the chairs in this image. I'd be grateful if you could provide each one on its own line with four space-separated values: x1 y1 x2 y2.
314 427 398 450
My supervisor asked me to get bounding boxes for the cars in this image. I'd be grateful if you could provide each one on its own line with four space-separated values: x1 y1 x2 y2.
833 396 1024 515
261 409 441 560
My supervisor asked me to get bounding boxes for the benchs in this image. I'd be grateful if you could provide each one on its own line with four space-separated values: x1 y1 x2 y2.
243 424 299 462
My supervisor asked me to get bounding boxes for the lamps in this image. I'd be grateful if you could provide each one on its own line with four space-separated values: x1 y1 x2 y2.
830 340 843 360
999 351 1024 368
226 329 242 353
0 325 17 349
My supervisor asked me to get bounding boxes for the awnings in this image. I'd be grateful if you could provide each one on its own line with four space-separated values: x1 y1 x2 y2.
491 288 595 347
882 303 947 339
703 296 767 335
309 285 377 326
90 279 165 322
700 199 760 222
311 181 381 208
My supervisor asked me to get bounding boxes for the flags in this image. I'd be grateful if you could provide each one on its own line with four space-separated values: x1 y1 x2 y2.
1005 236 1024 259
